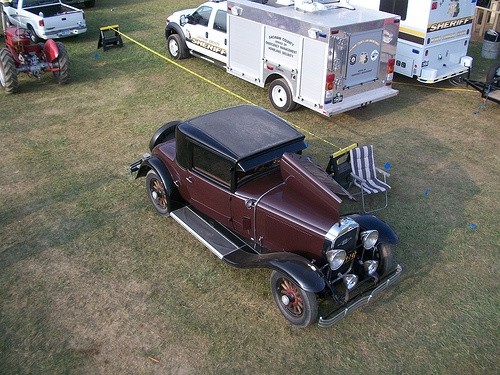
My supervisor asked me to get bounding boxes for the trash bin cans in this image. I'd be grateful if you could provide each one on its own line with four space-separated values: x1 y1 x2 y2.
481 30 500 59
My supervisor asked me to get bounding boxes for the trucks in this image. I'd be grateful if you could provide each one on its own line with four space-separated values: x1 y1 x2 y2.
163 0 401 118
341 0 477 86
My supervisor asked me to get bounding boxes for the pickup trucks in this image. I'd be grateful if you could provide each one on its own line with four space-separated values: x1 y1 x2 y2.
0 0 87 44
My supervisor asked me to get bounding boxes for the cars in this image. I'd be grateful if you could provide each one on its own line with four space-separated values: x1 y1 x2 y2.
131 104 404 332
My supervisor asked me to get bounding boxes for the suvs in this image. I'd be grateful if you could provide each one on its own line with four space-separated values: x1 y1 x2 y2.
0 0 71 95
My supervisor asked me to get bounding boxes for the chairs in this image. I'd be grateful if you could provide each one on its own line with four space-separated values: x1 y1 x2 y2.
347 145 391 214
